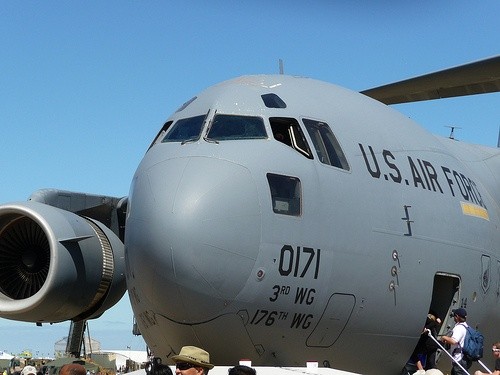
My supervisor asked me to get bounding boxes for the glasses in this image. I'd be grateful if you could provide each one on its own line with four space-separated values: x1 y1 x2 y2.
176 362 201 370
454 313 457 317
491 348 500 351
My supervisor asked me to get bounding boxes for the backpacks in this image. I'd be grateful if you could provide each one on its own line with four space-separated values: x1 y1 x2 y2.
458 324 484 360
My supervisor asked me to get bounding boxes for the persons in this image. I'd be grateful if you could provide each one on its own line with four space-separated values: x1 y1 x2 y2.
0 346 256 375
473 341 500 375
424 368 444 375
415 310 441 375
440 308 471 375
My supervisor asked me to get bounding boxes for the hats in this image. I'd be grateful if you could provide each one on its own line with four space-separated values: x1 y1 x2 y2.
452 308 467 319
171 345 214 370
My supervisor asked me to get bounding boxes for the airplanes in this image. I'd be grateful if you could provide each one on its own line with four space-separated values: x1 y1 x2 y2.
0 53 499 375
